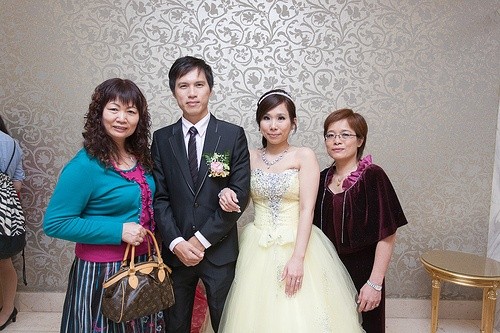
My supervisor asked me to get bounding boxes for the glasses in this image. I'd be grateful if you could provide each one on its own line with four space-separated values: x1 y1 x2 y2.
325 133 356 140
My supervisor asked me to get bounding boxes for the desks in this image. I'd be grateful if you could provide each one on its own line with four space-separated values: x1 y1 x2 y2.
420 251 500 333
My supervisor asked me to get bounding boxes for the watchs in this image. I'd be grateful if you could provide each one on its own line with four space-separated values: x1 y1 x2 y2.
367 280 382 291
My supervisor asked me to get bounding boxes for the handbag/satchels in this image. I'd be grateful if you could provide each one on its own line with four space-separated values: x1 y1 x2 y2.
101 229 175 323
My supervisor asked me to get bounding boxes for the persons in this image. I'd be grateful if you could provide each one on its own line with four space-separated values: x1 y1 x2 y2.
43 78 165 333
218 90 366 333
0 115 26 331
151 56 251 333
312 109 408 333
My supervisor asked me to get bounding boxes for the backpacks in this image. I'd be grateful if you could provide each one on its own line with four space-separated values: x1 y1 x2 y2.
0 141 26 259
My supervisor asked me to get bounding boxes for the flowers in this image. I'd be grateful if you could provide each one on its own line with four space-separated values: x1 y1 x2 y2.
202 151 230 178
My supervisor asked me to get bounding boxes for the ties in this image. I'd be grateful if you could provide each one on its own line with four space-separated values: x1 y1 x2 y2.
187 126 199 188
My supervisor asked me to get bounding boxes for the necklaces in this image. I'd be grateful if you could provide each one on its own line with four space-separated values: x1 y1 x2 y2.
262 146 289 166
336 164 357 186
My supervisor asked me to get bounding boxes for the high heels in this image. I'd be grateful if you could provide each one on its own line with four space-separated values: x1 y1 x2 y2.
0 307 18 331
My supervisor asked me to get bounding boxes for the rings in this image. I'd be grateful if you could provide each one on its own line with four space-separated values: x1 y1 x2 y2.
296 281 300 282
376 304 378 306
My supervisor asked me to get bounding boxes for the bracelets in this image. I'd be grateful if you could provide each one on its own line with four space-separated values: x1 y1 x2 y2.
218 188 230 198
